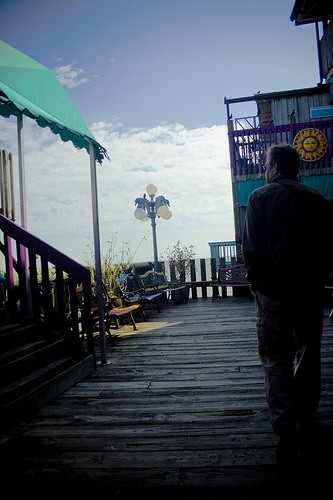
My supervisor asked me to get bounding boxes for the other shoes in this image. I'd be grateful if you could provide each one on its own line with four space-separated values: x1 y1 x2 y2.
277 431 299 460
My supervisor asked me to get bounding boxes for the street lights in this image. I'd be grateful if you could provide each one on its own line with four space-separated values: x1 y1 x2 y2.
133 183 172 284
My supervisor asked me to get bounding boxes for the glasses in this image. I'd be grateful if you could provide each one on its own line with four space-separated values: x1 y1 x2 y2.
263 162 274 170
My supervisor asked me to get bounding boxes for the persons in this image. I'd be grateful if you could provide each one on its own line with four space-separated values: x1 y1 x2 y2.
240 141 333 467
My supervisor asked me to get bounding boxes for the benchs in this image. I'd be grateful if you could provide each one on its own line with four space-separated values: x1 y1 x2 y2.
75 281 140 347
136 270 192 310
111 273 163 322
210 263 251 303
